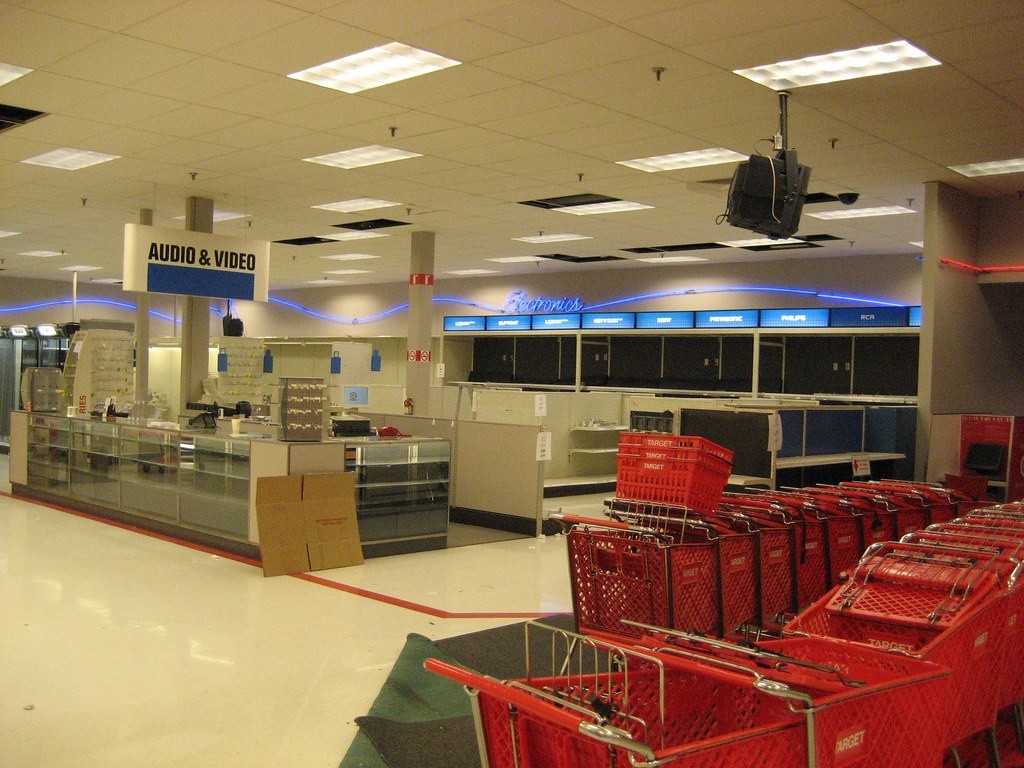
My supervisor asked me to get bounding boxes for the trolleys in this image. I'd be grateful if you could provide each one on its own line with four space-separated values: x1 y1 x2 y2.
420 477 1024 768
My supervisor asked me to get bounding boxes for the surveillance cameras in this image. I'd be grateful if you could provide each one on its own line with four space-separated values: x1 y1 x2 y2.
838 193 858 205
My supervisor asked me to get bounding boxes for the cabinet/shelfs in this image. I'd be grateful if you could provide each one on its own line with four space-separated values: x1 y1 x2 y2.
569 424 629 462
69 415 174 512
119 419 226 528
178 427 279 543
323 435 452 546
278 375 327 442
217 336 264 409
21 367 64 412
27 411 129 500
61 328 91 416
90 329 134 411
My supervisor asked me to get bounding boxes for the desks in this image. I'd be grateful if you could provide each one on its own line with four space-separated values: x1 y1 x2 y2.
776 452 907 481
936 476 1007 488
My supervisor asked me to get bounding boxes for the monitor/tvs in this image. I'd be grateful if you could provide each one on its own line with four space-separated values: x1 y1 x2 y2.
343 386 369 406
963 442 1005 481
